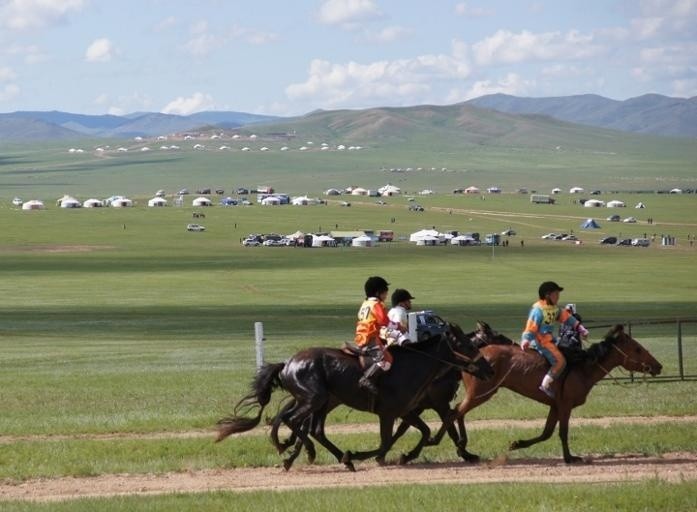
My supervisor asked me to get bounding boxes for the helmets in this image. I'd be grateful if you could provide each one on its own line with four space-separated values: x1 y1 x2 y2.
364 276 392 297
538 279 564 298
390 288 416 306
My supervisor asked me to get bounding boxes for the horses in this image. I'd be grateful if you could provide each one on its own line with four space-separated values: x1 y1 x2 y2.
213 320 521 473
422 323 663 464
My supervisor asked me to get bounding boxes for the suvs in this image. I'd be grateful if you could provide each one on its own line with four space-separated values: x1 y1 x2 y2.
243 239 259 247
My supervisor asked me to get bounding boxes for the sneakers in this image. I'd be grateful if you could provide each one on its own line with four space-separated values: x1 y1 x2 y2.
539 384 559 400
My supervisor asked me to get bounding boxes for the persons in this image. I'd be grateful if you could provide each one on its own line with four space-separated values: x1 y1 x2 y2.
553 303 583 350
520 282 589 400
354 276 407 394
379 288 414 345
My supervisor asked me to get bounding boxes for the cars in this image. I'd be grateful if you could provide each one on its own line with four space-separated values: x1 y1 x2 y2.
502 230 516 235
12 199 22 205
624 217 637 223
177 187 248 195
187 224 205 231
601 237 617 244
607 215 619 221
409 204 424 212
155 190 165 196
263 239 296 246
542 233 575 241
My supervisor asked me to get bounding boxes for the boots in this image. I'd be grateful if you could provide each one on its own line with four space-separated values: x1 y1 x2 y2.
357 362 385 396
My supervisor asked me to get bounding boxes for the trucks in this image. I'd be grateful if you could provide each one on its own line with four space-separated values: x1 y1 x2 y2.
531 194 555 204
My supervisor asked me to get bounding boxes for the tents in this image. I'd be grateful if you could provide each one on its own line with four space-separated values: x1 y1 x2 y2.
21 199 44 210
56 195 133 208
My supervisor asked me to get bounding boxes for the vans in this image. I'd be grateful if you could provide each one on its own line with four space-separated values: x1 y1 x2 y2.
632 238 650 247
